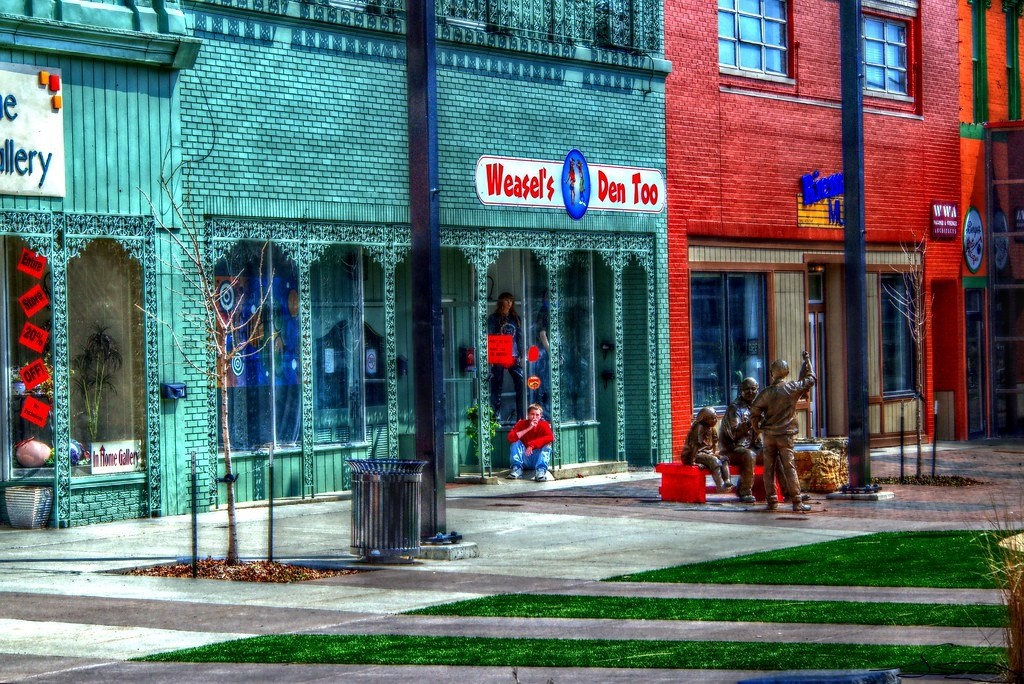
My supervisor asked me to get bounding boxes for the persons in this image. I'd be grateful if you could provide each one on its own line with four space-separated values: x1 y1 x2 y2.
680 405 735 494
487 291 524 422
719 376 812 502
536 290 551 420
749 349 819 511
506 403 554 482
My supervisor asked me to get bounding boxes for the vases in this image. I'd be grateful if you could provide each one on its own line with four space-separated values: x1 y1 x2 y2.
5 485 54 530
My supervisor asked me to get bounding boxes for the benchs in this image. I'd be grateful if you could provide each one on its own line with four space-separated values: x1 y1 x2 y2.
656 462 784 504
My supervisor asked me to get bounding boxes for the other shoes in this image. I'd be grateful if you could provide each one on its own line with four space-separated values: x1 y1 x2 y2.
535 469 546 481
506 468 523 479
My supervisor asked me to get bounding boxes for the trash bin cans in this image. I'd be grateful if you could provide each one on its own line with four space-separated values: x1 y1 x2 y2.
344 459 428 563
444 432 460 482
398 434 417 461
